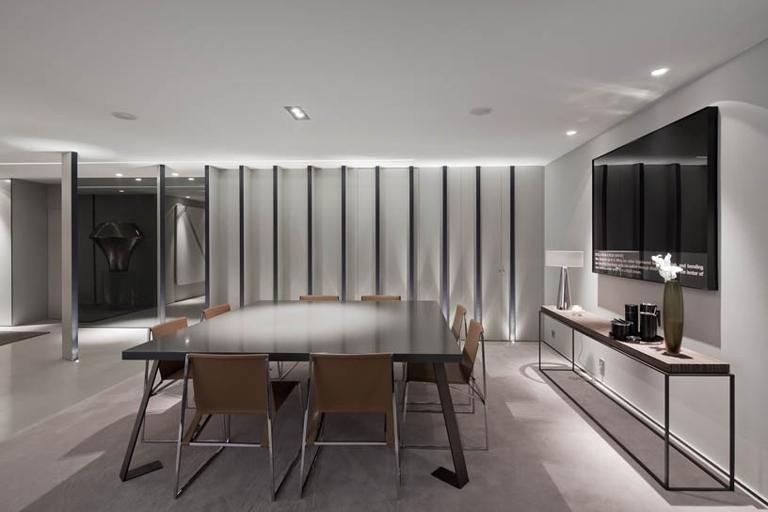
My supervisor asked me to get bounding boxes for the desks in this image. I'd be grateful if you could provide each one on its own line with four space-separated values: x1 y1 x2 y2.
538 304 735 493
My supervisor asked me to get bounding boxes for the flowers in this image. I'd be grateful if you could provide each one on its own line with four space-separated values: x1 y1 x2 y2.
650 252 683 283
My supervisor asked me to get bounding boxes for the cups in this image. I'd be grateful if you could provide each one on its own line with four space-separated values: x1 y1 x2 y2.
610 302 660 340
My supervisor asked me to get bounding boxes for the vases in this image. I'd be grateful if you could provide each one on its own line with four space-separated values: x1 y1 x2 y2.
662 280 685 354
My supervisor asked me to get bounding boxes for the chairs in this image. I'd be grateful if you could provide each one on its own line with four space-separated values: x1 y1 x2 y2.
140 304 299 443
299 353 403 500
173 352 305 501
299 295 488 447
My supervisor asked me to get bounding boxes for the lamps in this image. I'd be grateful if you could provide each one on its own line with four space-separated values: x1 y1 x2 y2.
544 250 585 309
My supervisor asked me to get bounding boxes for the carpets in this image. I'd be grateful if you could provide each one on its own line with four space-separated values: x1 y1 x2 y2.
0 330 50 346
